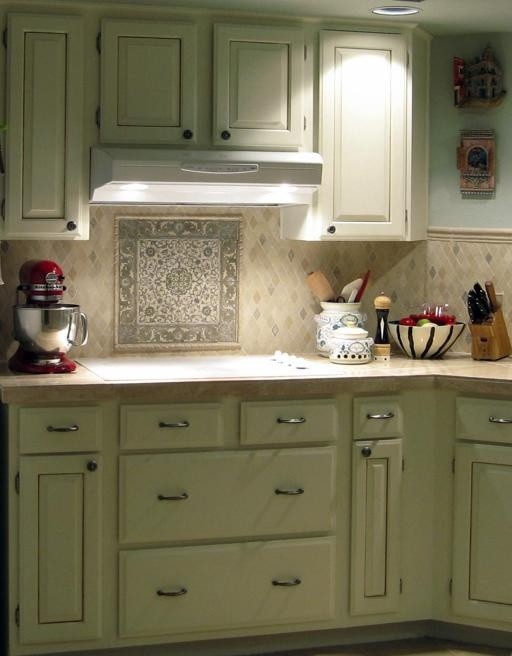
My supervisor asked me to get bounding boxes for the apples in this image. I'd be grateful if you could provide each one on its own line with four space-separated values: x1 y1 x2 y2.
400 314 456 327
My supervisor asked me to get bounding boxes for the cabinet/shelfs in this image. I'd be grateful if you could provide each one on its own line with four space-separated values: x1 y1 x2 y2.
451 393 511 625
15 405 107 644
283 18 432 242
96 18 307 151
0 0 90 242
119 397 338 639
350 396 406 618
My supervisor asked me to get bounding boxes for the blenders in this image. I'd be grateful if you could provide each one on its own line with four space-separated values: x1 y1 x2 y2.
8 258 89 374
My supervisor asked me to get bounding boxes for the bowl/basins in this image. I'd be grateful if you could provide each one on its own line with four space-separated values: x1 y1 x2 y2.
386 319 466 360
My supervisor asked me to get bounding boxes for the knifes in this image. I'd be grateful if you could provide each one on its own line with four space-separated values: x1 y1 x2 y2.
467 281 499 325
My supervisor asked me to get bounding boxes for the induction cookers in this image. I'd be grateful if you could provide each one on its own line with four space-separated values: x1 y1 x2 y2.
73 350 346 381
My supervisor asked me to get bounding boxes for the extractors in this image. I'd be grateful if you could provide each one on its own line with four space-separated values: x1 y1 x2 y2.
90 146 324 209
